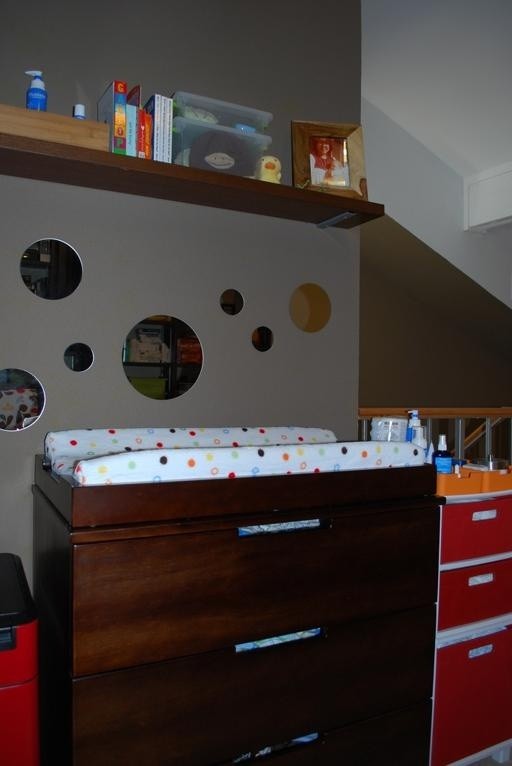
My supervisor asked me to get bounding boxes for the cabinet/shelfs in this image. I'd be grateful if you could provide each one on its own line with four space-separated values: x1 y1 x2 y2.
27 484 446 765
429 488 511 764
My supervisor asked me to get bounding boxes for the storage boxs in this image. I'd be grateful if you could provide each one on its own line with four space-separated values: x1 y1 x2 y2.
0 85 275 181
2 551 41 763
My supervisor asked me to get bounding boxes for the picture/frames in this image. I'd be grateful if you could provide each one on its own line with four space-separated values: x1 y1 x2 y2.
292 120 370 202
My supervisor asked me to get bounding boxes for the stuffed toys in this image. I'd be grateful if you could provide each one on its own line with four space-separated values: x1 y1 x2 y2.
244 154 282 184
173 130 258 178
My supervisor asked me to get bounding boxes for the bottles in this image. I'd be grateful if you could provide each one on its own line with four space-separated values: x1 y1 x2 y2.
73 104 86 120
488 453 496 470
432 435 452 474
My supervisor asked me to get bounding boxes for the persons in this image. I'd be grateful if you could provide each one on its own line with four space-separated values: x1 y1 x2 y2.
310 137 348 186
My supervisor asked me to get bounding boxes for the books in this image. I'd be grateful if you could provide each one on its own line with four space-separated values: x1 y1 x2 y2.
97 76 174 163
